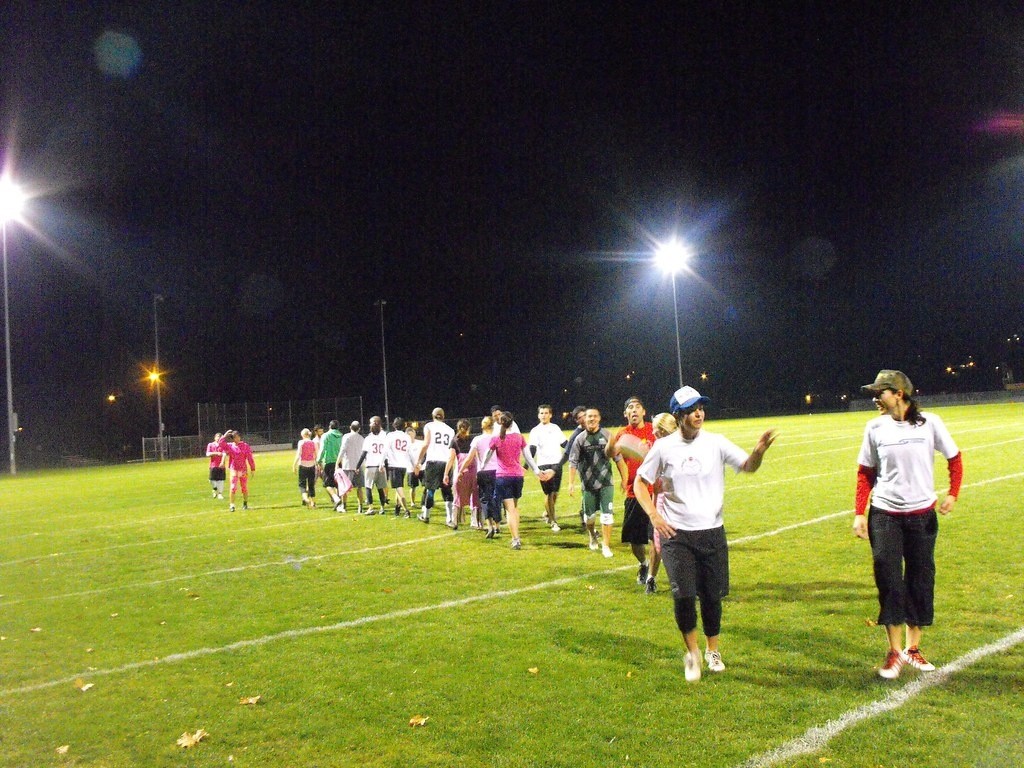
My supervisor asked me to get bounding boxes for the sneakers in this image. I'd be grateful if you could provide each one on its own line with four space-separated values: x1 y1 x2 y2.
684 651 704 683
903 645 936 672
879 646 905 679
704 650 726 671
637 561 658 597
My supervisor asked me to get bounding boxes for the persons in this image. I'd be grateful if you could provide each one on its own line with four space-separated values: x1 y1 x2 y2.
634 387 776 683
293 396 680 593
853 370 963 679
205 429 255 512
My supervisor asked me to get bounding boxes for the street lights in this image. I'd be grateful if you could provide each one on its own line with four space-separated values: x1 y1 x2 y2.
0 193 16 474
668 251 683 388
373 299 390 432
154 294 165 461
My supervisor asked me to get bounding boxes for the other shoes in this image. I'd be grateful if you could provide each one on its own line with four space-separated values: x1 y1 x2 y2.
543 509 561 532
230 504 235 512
589 530 599 550
212 492 224 500
445 520 508 539
418 513 429 523
243 501 247 510
602 545 613 558
511 537 522 550
301 496 417 519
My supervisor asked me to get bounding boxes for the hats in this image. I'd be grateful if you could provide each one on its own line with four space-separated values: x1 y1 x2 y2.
432 407 444 419
670 385 710 414
860 370 915 397
